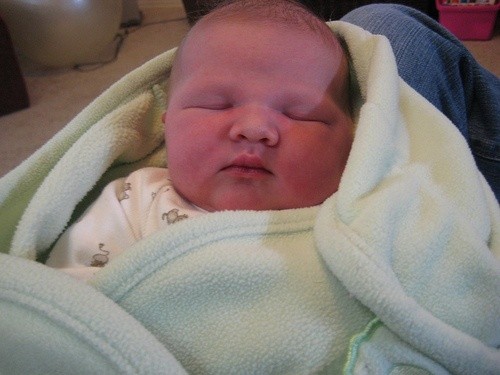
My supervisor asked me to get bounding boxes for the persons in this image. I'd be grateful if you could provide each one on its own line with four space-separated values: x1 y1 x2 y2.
0 0 500 374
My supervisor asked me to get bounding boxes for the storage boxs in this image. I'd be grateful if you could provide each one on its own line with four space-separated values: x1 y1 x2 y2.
435 0 500 41
